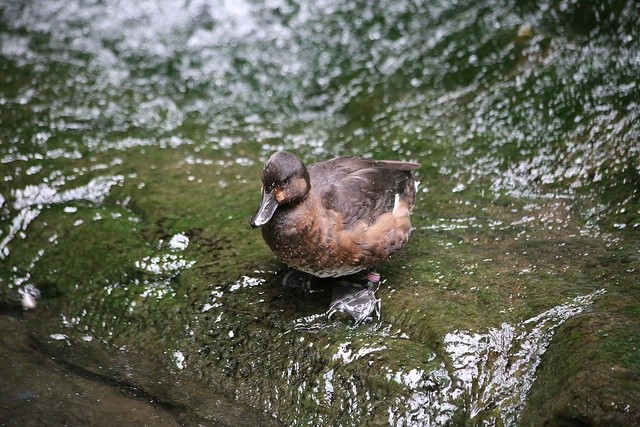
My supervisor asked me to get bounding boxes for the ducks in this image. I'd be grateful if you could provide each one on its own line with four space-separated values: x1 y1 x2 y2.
248 150 422 328
